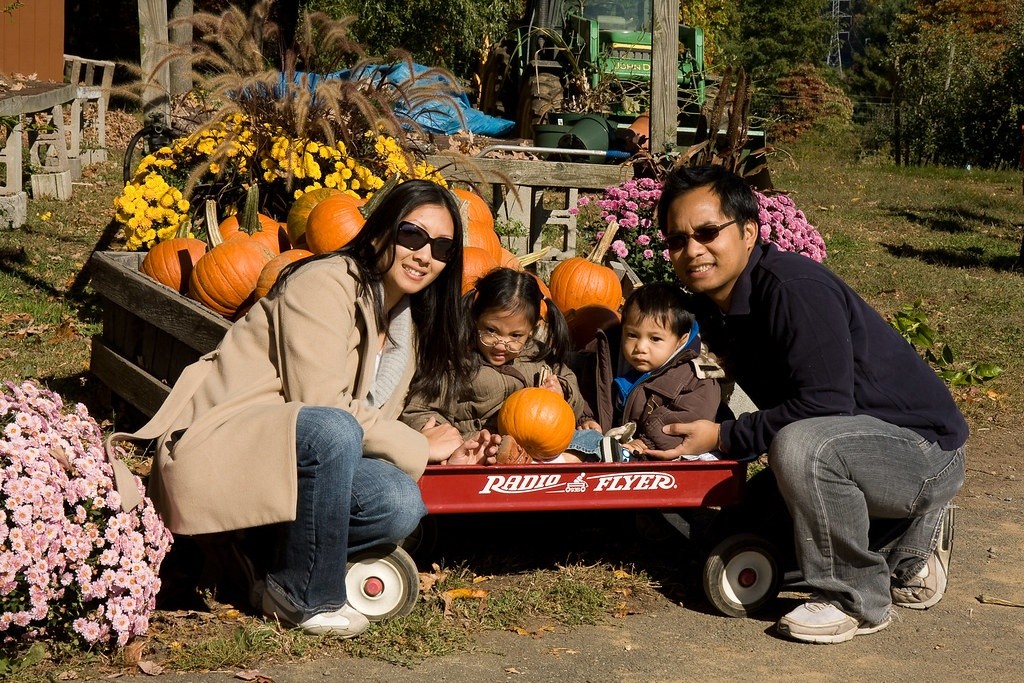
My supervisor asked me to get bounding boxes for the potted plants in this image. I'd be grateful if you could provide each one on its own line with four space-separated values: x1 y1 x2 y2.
496 24 624 124
624 97 682 153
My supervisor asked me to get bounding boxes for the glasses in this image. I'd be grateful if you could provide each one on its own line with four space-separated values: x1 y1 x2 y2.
474 321 527 354
395 221 457 264
665 219 737 252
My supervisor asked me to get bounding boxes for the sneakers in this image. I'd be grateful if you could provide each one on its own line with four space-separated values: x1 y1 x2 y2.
891 502 956 608
777 601 891 645
262 587 369 639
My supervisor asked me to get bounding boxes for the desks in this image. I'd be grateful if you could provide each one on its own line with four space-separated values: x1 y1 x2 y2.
0 81 76 228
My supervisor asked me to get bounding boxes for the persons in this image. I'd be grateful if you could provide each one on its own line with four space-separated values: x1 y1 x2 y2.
398 266 724 466
104 179 503 640
641 163 971 645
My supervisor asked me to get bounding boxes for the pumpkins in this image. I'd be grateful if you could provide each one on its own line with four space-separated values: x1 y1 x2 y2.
139 175 398 318
445 186 623 346
499 387 575 460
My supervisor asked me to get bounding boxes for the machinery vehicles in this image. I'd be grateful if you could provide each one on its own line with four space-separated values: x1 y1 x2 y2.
470 0 789 198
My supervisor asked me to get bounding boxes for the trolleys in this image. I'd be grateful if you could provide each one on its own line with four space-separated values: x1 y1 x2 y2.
342 406 786 617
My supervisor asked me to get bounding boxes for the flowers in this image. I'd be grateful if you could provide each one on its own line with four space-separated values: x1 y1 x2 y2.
568 177 826 294
113 86 449 251
0 379 174 648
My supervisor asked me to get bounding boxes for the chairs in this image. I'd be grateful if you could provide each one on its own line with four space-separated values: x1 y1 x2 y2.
63 55 116 156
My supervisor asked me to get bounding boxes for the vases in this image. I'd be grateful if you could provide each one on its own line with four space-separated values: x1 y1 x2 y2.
559 115 615 163
531 123 571 161
